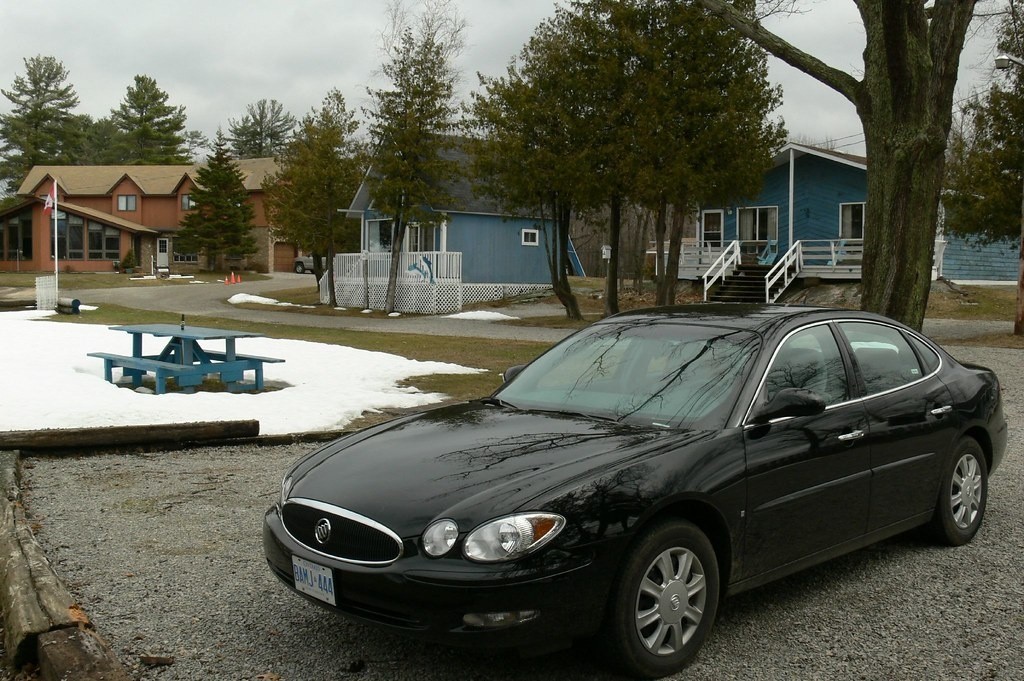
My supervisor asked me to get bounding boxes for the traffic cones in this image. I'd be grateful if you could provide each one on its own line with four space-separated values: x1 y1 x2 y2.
236 274 241 283
224 276 229 285
230 272 236 284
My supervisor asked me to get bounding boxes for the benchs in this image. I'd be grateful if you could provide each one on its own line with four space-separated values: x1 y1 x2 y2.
86 351 196 395
203 350 286 390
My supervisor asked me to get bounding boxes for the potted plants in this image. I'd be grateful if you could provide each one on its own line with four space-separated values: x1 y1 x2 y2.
121 248 136 274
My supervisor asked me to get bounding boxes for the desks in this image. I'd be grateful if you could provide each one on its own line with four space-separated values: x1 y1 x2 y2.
109 324 267 394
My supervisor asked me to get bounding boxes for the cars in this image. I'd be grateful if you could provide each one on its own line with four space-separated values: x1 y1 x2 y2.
260 301 1007 681
294 251 335 274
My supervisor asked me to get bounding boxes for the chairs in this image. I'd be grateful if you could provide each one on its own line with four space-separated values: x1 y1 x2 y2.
854 347 902 390
778 347 832 404
